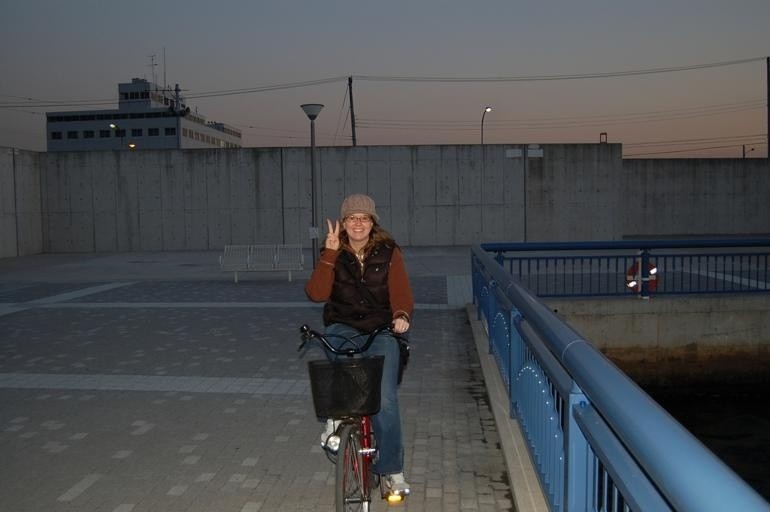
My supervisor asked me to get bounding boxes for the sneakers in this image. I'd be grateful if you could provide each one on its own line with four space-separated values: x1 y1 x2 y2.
384 473 410 495
320 418 343 451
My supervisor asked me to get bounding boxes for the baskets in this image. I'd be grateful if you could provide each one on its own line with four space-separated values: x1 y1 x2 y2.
307 355 385 417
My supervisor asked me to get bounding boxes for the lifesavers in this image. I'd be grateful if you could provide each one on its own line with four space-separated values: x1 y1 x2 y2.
626 261 658 292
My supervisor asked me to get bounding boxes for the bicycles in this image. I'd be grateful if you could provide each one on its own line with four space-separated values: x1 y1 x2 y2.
288 322 411 512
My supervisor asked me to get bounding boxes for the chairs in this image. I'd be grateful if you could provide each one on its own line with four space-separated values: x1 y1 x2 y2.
217 243 305 283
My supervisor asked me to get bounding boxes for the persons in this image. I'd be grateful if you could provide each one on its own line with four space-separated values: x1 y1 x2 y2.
304 194 414 494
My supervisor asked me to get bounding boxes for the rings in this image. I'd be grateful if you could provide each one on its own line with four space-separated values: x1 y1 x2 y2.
402 328 406 330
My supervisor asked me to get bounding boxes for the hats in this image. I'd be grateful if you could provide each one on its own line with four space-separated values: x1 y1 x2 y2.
341 194 379 221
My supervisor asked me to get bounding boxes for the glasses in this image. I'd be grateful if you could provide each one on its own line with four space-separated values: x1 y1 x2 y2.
344 214 370 222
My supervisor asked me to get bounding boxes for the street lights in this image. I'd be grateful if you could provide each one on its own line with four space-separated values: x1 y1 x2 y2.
108 121 124 148
742 144 755 158
299 102 326 273
479 105 493 144
